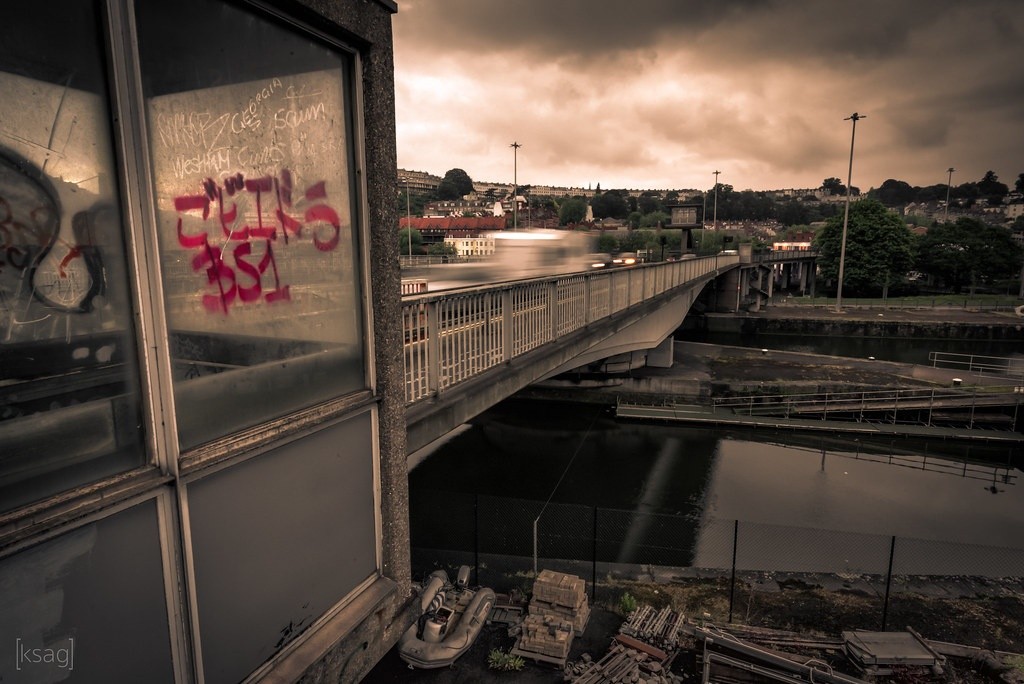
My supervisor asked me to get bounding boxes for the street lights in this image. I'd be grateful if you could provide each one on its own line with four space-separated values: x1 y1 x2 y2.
702 191 708 249
944 165 957 222
711 170 723 247
403 174 413 262
510 142 523 229
836 111 867 311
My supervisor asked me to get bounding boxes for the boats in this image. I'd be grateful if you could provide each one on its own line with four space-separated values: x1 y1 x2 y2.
398 564 497 669
